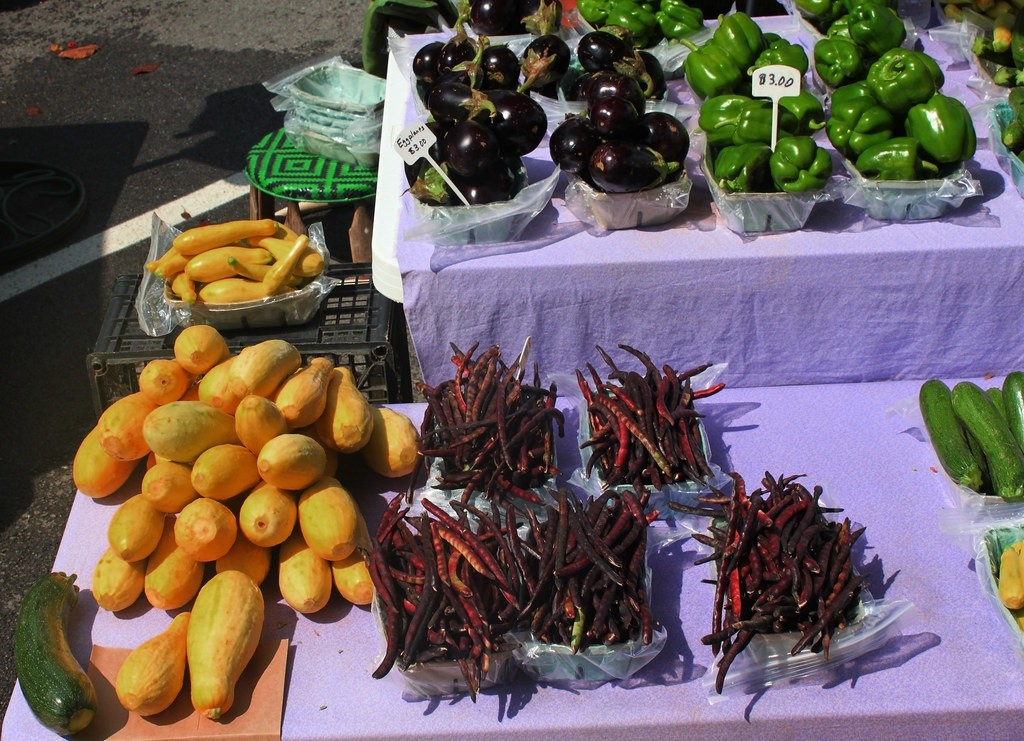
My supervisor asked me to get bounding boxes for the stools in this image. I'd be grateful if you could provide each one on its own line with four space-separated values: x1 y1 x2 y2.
244 125 379 279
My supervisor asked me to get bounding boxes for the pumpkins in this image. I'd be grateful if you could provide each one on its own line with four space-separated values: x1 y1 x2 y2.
16 219 421 734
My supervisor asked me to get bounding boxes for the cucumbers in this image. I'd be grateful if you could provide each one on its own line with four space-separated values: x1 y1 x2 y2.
918 370 1024 498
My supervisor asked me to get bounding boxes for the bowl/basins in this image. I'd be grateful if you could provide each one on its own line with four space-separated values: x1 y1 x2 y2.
702 135 825 234
532 90 588 137
708 517 866 663
285 64 386 167
566 172 690 229
585 391 709 497
426 454 559 522
370 586 512 697
410 155 528 245
917 383 1009 505
162 240 329 332
846 159 965 221
987 101 1024 198
981 524 1024 645
928 22 975 63
511 628 643 684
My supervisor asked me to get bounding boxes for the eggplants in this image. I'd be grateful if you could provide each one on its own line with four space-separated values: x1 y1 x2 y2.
404 32 690 206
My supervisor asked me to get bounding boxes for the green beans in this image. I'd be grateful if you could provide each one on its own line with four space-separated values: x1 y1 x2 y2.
358 337 867 701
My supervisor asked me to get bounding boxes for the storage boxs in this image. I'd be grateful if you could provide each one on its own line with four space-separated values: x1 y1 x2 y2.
413 195 516 244
584 388 711 519
285 63 386 166
931 445 1024 523
567 165 691 230
990 101 1024 199
522 570 650 682
433 433 560 524
84 262 412 422
711 518 865 666
702 154 825 234
373 589 508 697
979 523 1024 649
839 153 965 221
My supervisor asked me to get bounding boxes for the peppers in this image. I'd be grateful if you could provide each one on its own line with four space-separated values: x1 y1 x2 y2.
579 0 976 196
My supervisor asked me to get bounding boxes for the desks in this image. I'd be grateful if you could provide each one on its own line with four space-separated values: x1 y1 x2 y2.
399 17 1024 384
1 371 1024 741
